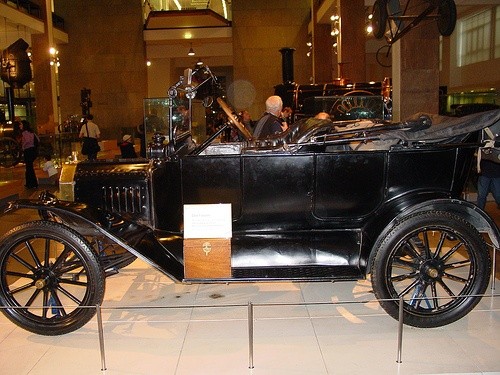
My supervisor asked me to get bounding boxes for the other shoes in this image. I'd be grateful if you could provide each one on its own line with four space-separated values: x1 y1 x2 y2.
24 183 39 188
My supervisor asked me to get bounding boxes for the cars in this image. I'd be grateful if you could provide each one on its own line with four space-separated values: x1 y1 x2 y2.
0 76 499 337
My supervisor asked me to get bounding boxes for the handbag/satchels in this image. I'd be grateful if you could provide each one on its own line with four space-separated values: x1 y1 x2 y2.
81 139 89 155
34 134 38 145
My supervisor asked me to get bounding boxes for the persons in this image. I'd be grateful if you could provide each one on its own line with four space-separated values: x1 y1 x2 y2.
120 135 137 158
42 154 58 187
19 120 40 188
476 159 500 210
137 96 333 158
79 115 102 160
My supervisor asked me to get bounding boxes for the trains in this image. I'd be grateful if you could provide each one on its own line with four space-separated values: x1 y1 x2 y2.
273 46 451 124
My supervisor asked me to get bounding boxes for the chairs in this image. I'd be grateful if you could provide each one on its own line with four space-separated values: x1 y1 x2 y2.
241 117 333 154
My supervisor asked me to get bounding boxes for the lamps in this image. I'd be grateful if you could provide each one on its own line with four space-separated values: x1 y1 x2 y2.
188 42 195 56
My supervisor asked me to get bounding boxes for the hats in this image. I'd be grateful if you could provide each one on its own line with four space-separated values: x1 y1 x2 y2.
86 115 94 120
123 135 132 141
